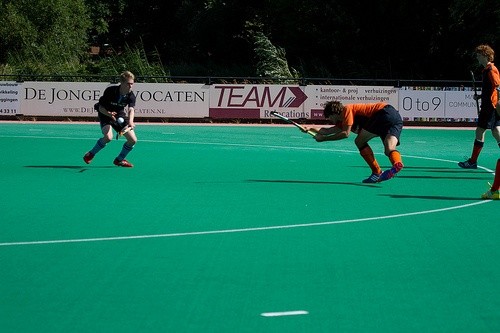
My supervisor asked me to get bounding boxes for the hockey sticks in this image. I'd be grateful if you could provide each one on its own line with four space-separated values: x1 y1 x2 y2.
116 124 135 140
469 71 480 118
270 111 317 136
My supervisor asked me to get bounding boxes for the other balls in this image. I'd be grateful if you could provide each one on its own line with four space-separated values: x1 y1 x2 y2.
118 117 124 123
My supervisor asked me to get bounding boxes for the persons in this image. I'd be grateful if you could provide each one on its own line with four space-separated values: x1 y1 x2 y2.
83 71 137 167
480 159 500 199
458 45 500 169
301 101 403 184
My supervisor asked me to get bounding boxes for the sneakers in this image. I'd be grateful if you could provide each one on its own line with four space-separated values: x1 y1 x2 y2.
379 161 403 180
459 160 477 169
481 186 500 199
363 171 385 183
114 157 133 166
83 151 93 164
